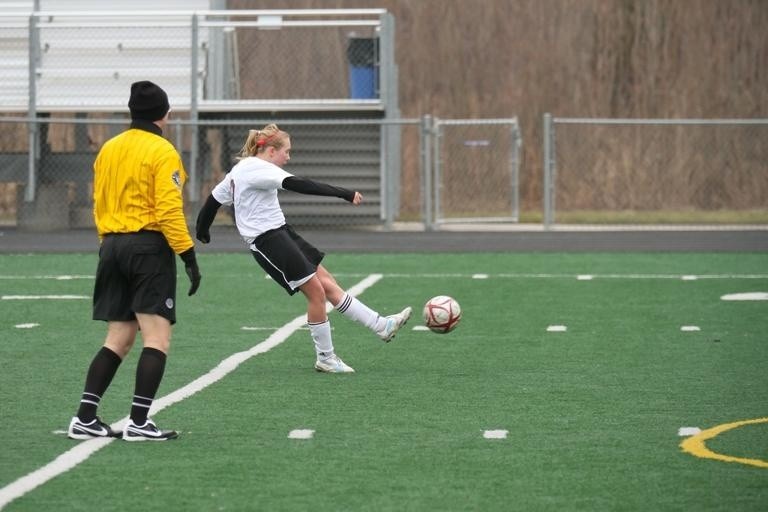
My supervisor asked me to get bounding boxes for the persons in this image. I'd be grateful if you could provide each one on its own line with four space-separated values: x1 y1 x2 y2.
66 79 203 443
193 120 412 375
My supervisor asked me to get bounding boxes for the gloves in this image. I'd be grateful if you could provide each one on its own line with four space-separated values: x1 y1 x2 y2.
184 261 202 297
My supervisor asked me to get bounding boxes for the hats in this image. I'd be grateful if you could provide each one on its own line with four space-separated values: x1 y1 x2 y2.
127 80 169 124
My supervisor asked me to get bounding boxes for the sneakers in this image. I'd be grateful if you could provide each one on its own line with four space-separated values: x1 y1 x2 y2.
314 353 356 374
67 415 123 439
121 418 180 441
376 306 413 345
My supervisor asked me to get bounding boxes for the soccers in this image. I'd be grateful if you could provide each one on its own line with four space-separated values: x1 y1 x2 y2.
423 295 461 333
